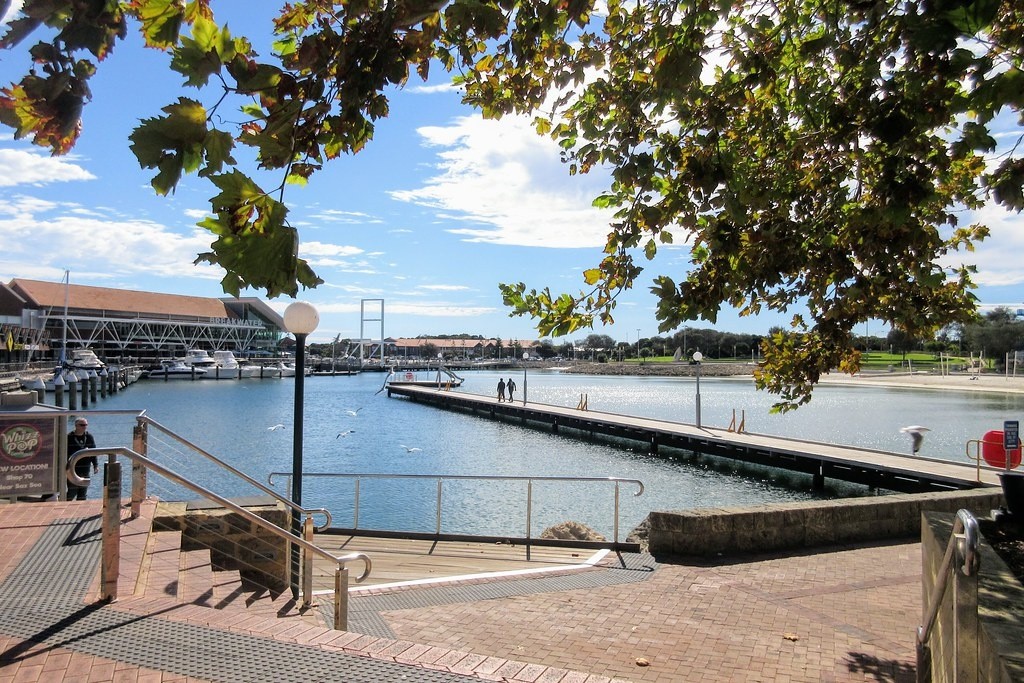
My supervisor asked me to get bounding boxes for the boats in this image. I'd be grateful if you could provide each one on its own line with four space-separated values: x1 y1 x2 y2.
146 347 312 379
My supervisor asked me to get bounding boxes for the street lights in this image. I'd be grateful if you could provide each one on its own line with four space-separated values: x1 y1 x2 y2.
693 351 702 428
438 353 443 391
522 353 529 407
283 302 321 594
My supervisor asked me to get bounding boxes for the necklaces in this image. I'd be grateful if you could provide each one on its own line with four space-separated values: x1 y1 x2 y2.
74 432 86 448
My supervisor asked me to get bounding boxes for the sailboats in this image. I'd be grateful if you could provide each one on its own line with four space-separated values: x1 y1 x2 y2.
15 267 126 392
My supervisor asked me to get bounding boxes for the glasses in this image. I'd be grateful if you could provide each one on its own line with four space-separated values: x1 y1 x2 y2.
77 424 87 426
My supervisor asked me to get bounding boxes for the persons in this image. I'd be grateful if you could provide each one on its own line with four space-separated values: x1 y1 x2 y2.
506 378 516 402
496 378 505 402
66 417 99 501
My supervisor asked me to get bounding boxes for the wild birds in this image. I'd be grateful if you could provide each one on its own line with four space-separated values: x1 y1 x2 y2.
347 407 363 417
899 425 932 456
336 430 356 439
400 445 423 453
268 424 286 432
